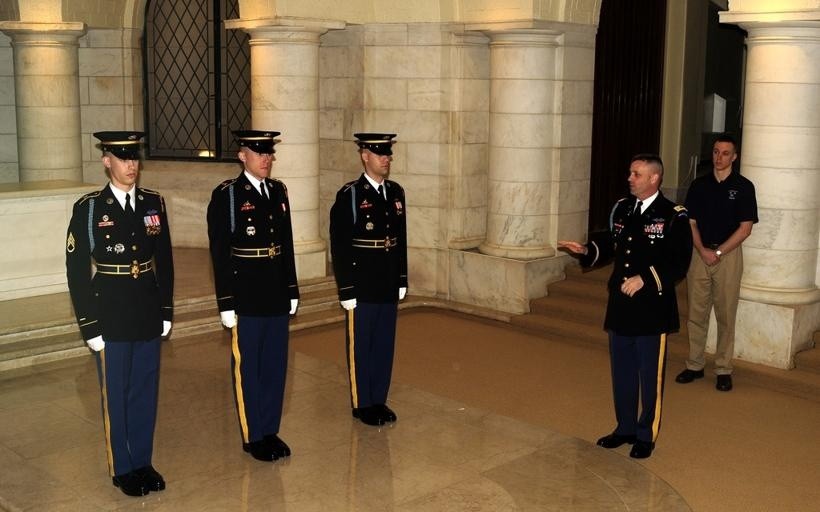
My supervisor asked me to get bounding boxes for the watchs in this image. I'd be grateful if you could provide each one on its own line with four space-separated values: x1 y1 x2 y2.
714 249 722 258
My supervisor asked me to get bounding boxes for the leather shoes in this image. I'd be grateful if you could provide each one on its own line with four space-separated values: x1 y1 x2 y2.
243 434 291 462
597 432 633 448
676 369 704 383
716 375 731 390
630 442 655 458
352 404 396 426
113 465 165 497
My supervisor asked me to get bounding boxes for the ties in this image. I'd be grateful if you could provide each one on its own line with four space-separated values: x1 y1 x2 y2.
634 201 643 216
378 185 385 200
125 193 133 213
260 182 268 199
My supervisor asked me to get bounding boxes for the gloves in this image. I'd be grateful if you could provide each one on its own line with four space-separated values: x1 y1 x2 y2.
220 310 235 328
399 287 407 300
289 299 298 314
340 298 357 310
87 335 105 352
161 321 171 336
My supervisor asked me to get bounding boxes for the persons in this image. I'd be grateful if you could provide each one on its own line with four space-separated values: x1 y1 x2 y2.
207 131 301 460
66 131 174 496
330 133 408 425
556 154 693 458
675 135 759 391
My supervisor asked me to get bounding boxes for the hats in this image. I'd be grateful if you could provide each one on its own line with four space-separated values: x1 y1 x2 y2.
93 131 145 160
354 133 396 155
233 130 280 153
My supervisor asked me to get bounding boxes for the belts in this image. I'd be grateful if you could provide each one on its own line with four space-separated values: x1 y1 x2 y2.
232 245 281 259
352 237 397 251
96 260 152 279
